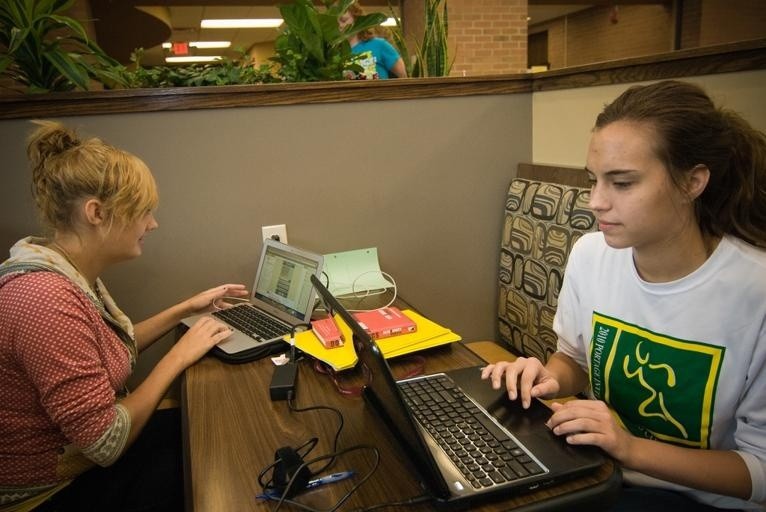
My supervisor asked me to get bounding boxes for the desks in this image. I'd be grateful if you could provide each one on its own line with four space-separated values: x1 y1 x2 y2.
179 290 622 511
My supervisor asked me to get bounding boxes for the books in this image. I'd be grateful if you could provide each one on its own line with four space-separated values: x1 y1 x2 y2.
310 306 417 348
282 309 464 374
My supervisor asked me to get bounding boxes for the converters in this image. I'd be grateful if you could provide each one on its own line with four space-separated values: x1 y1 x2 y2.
268 363 299 402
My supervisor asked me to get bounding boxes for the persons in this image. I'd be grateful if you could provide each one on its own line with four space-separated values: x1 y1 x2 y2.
478 79 766 510
333 0 409 80
0 116 252 511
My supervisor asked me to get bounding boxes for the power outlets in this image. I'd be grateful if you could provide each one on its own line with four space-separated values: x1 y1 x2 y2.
262 224 288 246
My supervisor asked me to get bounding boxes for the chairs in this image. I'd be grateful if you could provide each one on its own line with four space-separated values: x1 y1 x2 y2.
463 164 602 411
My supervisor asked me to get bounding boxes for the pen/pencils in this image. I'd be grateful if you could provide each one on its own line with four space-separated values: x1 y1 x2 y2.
256 470 355 499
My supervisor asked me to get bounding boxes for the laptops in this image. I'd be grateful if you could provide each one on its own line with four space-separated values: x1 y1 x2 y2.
179 237 324 356
309 273 607 508
